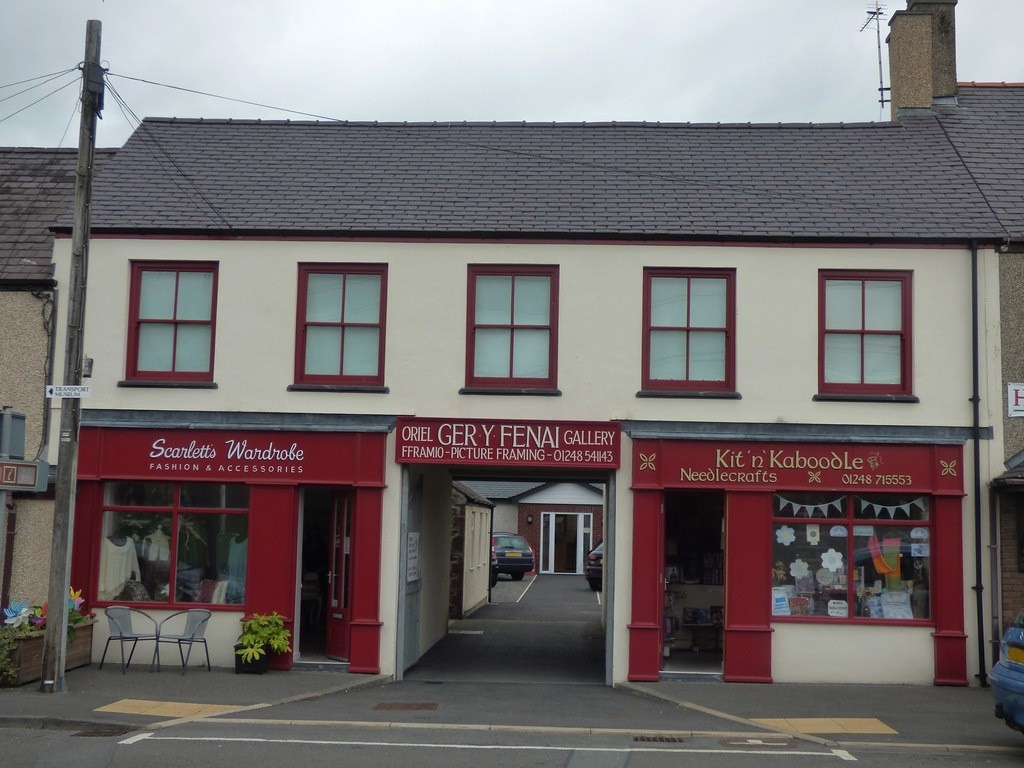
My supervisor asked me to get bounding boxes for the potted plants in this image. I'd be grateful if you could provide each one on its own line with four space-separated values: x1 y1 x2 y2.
233 611 291 672
0 618 100 688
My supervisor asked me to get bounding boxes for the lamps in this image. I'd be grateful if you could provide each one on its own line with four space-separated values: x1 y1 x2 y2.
528 514 533 525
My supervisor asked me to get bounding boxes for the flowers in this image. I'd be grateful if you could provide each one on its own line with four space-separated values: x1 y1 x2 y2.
0 590 98 640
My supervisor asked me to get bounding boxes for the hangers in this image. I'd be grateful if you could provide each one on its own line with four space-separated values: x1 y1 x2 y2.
106 518 170 540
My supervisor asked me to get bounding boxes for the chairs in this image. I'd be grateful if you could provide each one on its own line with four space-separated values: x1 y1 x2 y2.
98 605 160 674
148 608 213 675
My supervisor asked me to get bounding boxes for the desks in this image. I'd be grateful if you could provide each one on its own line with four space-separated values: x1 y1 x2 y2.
683 621 722 651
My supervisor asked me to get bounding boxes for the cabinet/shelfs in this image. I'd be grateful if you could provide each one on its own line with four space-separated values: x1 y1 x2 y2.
660 566 685 657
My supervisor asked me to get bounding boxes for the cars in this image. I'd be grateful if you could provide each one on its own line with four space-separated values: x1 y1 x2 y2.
108 535 245 606
848 541 930 616
989 606 1024 734
585 542 603 592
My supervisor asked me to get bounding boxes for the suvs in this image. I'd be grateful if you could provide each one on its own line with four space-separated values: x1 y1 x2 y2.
491 532 536 582
